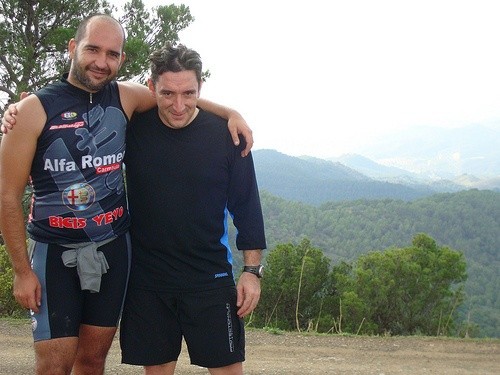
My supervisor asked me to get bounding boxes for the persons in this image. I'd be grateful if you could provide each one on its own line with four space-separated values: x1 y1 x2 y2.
0 47 264 374
0 12 255 374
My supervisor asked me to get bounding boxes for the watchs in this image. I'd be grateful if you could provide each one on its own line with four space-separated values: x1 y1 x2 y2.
242 264 264 279
